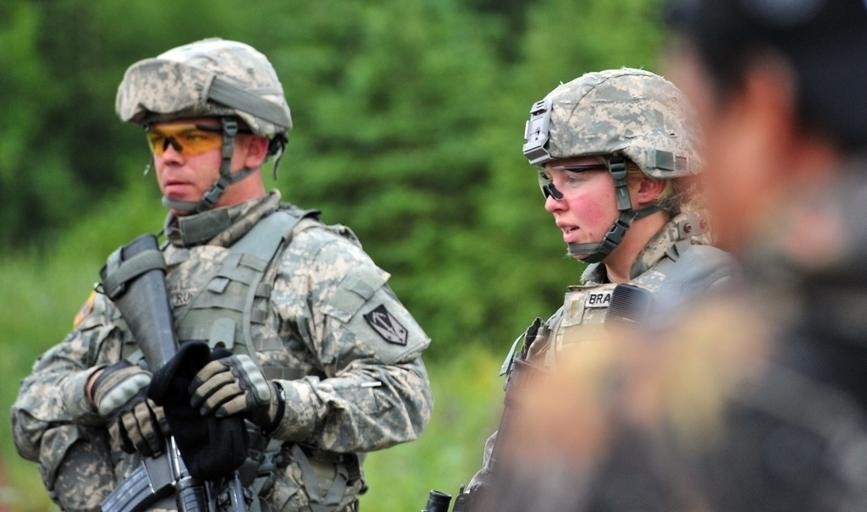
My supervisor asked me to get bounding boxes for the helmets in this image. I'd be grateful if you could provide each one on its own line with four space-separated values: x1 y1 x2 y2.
521 68 705 205
116 38 293 157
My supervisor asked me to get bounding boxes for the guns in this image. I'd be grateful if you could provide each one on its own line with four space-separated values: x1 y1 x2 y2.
94 233 271 512
422 360 549 511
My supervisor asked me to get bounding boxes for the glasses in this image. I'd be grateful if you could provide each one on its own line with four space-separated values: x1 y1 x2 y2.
142 122 253 159
536 164 609 201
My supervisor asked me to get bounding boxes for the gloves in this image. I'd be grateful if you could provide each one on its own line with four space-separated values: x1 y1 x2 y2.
89 358 173 460
185 348 286 433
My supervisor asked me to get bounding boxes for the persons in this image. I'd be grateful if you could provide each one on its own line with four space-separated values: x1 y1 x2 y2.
462 1 867 512
447 61 738 512
5 27 437 512
